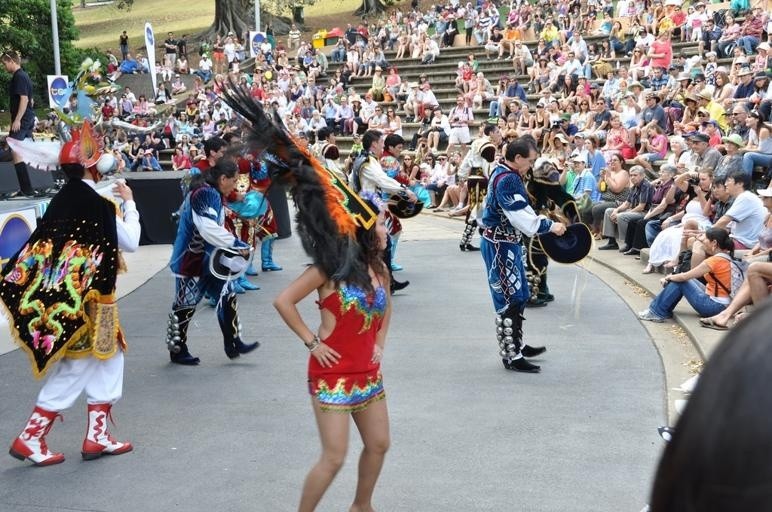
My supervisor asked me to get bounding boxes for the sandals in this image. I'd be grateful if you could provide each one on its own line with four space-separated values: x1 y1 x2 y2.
427 203 465 216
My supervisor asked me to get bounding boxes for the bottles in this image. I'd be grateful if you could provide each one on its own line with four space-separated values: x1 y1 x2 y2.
616 59 621 70
155 150 160 160
171 153 174 162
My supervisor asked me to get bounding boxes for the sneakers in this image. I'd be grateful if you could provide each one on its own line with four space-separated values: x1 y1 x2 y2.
624 248 640 255
638 311 665 324
7 191 36 201
619 246 632 252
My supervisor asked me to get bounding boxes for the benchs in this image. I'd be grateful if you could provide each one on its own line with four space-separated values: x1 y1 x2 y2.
152 31 772 265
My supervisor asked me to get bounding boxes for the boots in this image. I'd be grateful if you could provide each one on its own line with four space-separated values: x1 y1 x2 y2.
81 403 133 461
459 220 481 252
234 238 283 294
216 294 260 359
165 302 200 365
9 406 65 467
383 241 409 295
391 239 403 271
494 302 541 373
519 308 546 359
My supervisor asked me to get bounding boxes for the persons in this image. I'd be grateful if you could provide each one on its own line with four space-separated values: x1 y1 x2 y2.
274 201 391 512
166 161 260 363
649 298 772 512
478 140 566 373
8 156 140 465
35 1 772 328
1 49 36 200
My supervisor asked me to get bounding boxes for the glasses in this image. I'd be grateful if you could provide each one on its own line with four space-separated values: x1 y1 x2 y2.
405 157 445 162
507 135 516 138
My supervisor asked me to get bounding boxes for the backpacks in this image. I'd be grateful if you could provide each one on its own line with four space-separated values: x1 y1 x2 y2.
708 254 752 302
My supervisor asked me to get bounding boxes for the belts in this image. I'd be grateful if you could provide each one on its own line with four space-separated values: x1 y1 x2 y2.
451 125 464 128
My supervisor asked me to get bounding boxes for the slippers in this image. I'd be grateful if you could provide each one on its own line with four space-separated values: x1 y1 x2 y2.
698 317 728 330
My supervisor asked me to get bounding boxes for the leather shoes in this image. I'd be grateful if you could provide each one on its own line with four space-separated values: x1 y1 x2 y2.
643 265 655 274
663 262 679 268
527 300 544 307
598 242 619 250
538 293 554 302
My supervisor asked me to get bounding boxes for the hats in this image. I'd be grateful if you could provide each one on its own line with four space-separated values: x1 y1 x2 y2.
559 112 572 121
572 156 586 163
515 19 566 63
589 83 599 90
311 85 373 120
574 132 586 140
549 134 569 148
61 142 84 165
387 194 424 220
668 63 678 71
253 37 323 96
756 186 772 197
536 102 544 109
411 73 431 91
207 246 255 281
353 134 360 139
675 71 693 82
628 81 645 93
681 90 746 148
694 2 707 11
644 93 661 104
537 222 593 264
705 41 770 84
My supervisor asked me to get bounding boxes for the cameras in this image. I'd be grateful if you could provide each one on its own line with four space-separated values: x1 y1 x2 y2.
687 176 700 187
